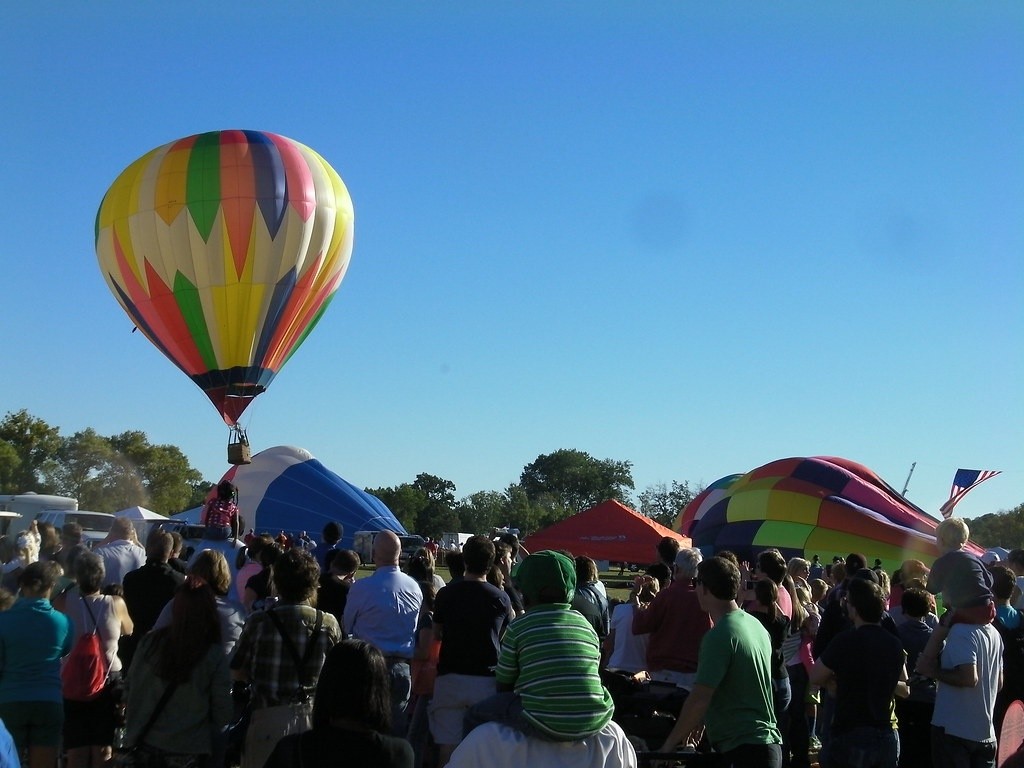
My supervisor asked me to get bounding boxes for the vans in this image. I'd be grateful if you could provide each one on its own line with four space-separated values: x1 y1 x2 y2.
30 508 116 550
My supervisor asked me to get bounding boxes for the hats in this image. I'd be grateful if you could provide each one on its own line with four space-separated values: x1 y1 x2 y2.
857 568 879 583
902 560 930 575
511 550 577 602
981 551 1001 565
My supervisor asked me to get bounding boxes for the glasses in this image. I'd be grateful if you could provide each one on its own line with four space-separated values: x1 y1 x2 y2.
692 577 702 589
840 596 848 607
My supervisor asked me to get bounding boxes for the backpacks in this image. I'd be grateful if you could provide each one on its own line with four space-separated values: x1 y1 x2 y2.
61 597 112 702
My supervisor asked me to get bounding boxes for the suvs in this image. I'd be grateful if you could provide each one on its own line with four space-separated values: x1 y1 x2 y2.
397 535 428 562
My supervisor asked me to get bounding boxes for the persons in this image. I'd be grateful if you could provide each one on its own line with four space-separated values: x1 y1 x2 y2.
657 557 784 768
264 639 415 768
463 550 616 743
0 480 1023 768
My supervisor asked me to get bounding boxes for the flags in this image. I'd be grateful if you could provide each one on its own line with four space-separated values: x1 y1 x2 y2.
939 469 1004 520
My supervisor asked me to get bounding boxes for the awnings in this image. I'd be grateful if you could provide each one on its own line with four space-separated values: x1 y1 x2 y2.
111 503 204 524
518 497 694 567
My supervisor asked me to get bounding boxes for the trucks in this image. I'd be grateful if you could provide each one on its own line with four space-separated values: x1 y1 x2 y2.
443 533 475 555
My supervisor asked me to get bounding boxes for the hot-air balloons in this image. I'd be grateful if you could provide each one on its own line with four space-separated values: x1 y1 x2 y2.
686 456 996 590
93 127 355 468
672 471 748 543
203 444 429 569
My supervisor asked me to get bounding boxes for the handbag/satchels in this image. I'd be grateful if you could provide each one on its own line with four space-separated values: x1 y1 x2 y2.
112 747 138 768
